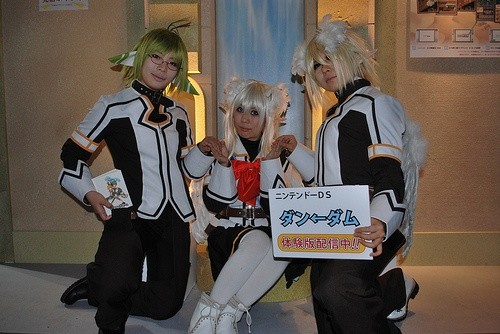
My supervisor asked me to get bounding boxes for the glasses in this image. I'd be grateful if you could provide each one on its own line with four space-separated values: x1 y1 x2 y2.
148 54 179 71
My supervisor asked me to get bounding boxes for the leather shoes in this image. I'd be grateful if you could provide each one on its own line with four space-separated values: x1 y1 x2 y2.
61 276 88 305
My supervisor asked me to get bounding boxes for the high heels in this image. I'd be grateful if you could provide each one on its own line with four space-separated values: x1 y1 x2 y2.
386 268 420 322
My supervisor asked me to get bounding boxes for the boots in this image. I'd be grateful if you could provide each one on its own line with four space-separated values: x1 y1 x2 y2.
186 290 226 334
215 293 247 334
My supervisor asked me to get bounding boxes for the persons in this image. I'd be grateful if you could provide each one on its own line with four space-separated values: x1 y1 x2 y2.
188 78 303 334
60 28 222 334
271 14 420 334
